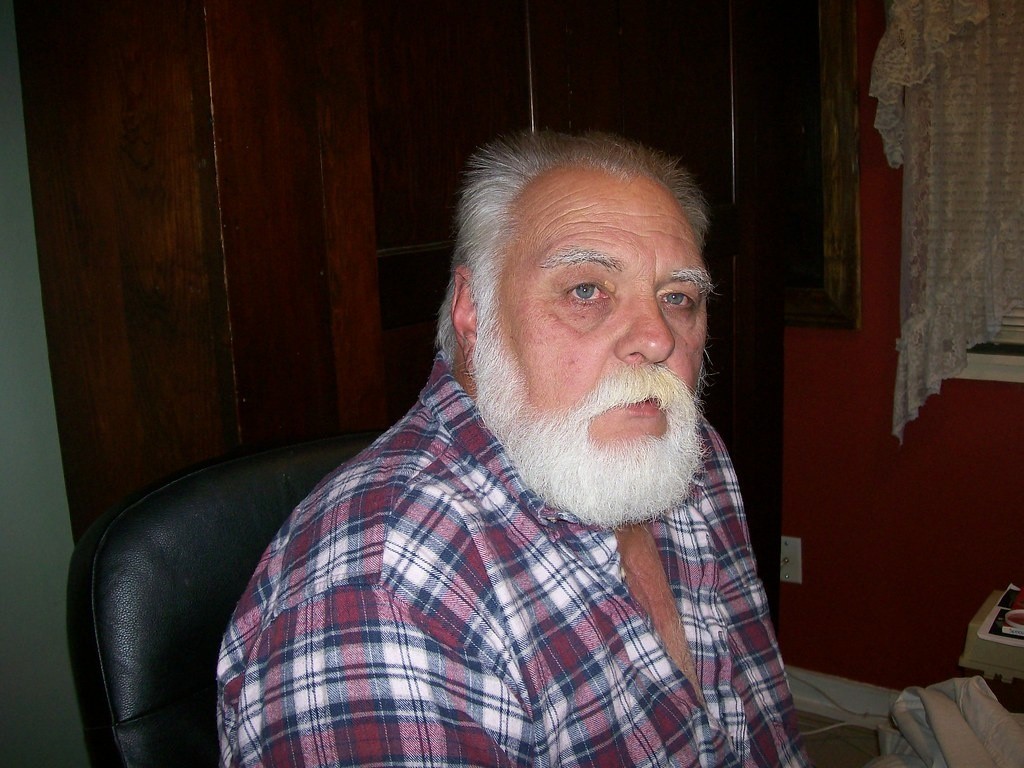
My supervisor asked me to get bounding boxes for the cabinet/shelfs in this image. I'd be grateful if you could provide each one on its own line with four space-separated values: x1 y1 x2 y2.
13 0 792 639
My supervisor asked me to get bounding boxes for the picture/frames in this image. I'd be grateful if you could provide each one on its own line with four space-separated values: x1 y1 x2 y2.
773 0 863 330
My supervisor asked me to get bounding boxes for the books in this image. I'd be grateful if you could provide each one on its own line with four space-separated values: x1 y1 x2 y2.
978 583 1024 647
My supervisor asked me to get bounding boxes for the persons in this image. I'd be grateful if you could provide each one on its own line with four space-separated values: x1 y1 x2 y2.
216 131 810 768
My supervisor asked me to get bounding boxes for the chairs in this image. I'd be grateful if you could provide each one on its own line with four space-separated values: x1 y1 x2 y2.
66 428 390 768
